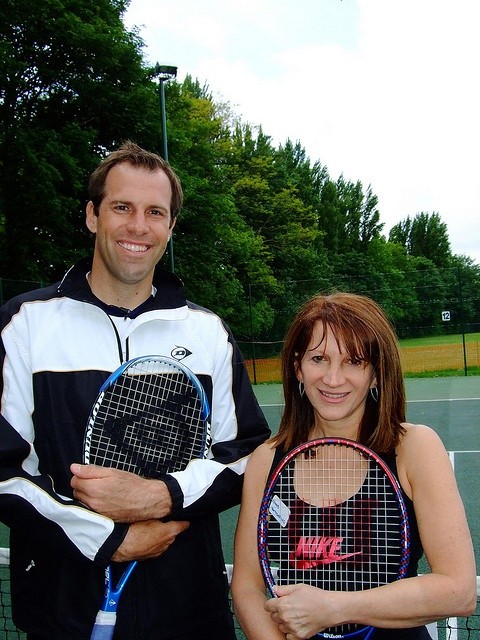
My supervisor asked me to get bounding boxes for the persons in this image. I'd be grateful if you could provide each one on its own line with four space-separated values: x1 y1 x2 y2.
0 138 272 640
230 292 479 639
